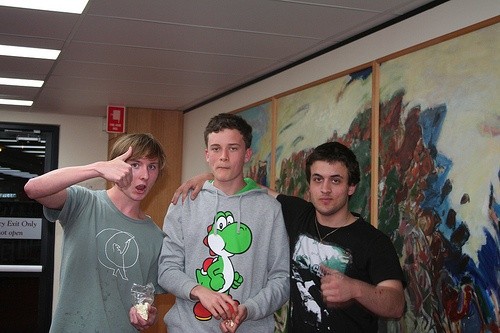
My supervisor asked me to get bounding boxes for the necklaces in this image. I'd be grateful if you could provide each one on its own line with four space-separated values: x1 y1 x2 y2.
310 214 353 245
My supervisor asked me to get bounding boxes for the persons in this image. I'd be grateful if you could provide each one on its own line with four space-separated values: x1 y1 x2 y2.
171 142 407 333
157 113 290 333
23 131 168 333
